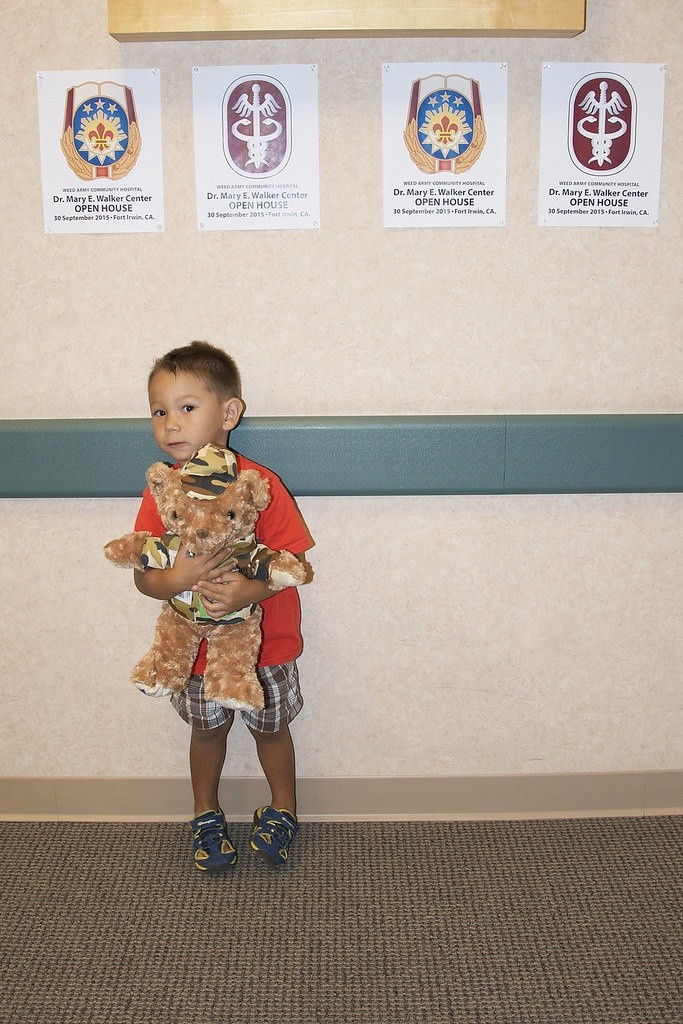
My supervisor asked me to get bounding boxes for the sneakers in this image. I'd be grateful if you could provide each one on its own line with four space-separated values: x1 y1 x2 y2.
187 803 234 873
247 803 300 865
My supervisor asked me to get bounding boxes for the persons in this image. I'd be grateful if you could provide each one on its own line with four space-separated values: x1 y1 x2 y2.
131 340 315 871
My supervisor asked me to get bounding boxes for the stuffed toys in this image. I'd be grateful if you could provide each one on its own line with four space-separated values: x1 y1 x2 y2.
100 441 311 709
